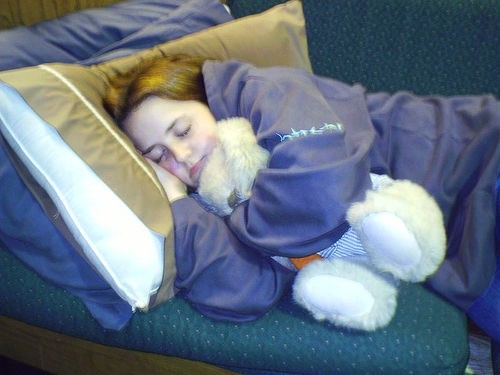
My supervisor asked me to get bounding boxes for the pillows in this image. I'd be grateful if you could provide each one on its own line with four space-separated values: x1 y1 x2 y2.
0 0 315 331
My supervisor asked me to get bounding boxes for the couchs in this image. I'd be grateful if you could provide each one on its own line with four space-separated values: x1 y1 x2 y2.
0 0 500 375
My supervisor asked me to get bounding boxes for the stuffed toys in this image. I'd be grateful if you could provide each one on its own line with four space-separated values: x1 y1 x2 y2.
196 117 445 333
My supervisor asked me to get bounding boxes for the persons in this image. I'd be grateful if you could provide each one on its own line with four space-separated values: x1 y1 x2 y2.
101 53 500 341
